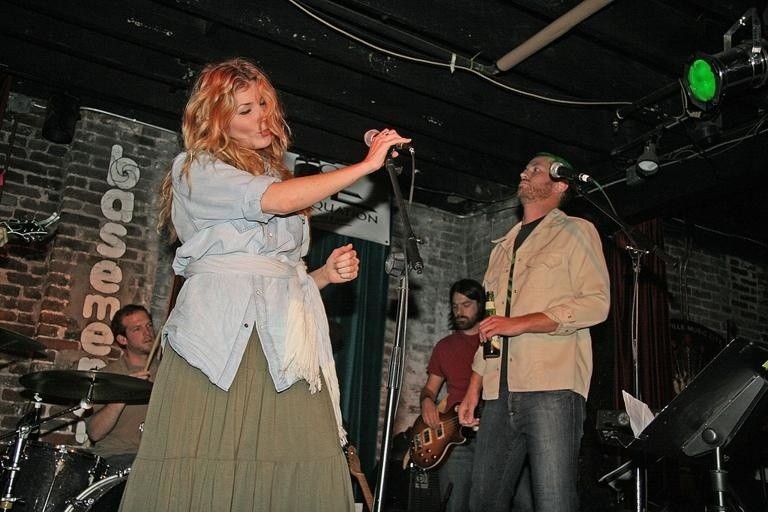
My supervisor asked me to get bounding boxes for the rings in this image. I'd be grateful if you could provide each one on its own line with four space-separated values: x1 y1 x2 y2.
383 131 388 136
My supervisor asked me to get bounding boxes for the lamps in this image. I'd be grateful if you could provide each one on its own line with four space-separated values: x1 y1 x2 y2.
676 9 768 114
636 125 662 173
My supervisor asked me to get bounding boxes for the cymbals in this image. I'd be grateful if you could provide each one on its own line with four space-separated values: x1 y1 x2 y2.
19 369 153 402
0 327 47 353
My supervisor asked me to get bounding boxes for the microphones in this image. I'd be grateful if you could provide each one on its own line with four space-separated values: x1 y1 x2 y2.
548 161 589 184
363 129 416 154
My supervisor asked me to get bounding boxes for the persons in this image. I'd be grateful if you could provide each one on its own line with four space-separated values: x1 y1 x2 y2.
456 150 610 512
420 279 534 512
119 58 412 511
86 305 164 474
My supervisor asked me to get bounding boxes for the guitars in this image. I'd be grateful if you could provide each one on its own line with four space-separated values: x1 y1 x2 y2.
348 445 375 510
409 401 484 472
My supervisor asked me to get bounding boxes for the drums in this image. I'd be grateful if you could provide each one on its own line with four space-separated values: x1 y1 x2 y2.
0 440 107 512
58 466 131 512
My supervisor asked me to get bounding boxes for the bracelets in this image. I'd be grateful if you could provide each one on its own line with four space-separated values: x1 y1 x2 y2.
420 395 434 402
